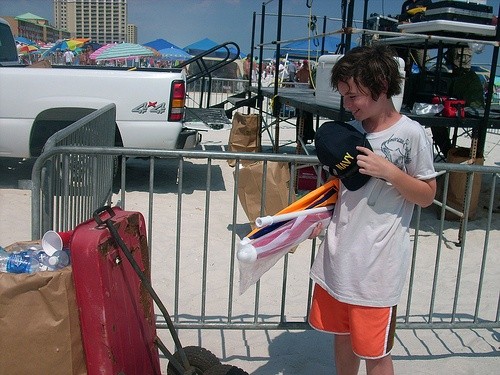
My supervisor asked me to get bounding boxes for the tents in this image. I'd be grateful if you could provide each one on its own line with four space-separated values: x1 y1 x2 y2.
274 33 364 59
141 38 247 78
15 37 102 63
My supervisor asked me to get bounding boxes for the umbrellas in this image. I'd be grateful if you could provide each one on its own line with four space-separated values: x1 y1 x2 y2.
90 42 194 70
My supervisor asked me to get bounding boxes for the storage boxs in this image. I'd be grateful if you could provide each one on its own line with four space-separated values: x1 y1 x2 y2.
434 97 465 116
314 54 408 107
424 1 494 23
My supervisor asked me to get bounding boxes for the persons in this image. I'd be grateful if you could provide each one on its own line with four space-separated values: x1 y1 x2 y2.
242 52 309 96
63 48 75 65
308 45 438 375
156 62 160 67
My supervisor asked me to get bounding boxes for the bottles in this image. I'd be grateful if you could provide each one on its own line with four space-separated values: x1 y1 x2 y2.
0 243 71 273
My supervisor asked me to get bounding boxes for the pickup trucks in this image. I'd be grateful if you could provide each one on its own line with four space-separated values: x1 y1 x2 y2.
0 17 202 196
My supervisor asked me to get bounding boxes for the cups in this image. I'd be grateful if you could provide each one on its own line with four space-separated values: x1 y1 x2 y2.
42 231 73 256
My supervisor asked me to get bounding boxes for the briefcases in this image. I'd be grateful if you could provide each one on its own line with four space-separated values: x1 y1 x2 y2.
432 92 465 118
425 0 494 25
315 53 406 115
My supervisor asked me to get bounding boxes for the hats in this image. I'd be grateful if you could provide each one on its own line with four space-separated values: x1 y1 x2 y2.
314 121 375 192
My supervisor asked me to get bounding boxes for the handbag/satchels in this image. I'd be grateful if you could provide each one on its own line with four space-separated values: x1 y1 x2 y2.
438 145 485 221
231 154 300 254
227 106 262 168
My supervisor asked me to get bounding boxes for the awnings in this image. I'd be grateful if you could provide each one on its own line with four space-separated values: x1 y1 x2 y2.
238 177 339 265
